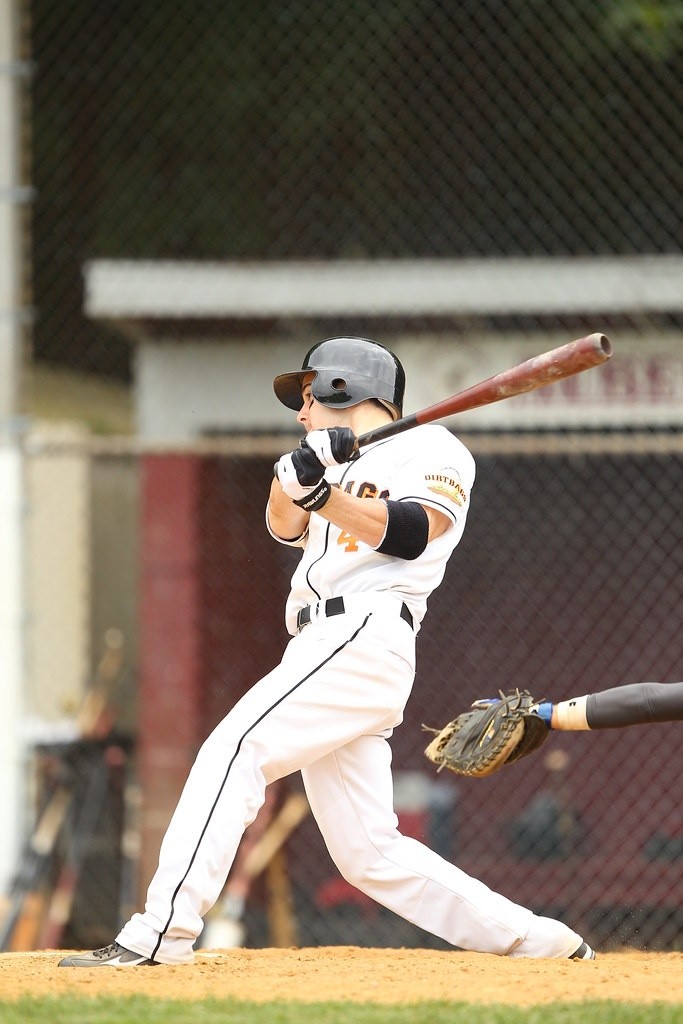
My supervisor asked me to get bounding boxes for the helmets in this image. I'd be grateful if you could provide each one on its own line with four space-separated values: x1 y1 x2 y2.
273 335 406 422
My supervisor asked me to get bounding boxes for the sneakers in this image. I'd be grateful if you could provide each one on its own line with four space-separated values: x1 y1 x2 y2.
568 939 595 961
56 940 167 968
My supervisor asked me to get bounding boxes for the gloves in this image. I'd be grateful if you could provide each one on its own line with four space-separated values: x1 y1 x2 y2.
274 440 331 513
304 426 360 468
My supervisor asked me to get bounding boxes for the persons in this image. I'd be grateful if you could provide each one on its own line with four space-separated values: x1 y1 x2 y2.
58 335 595 969
470 681 683 731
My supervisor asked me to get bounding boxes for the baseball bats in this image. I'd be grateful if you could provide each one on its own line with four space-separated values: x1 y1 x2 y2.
274 332 615 477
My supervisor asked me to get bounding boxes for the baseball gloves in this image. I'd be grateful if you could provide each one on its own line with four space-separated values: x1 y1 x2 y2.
424 688 551 778
